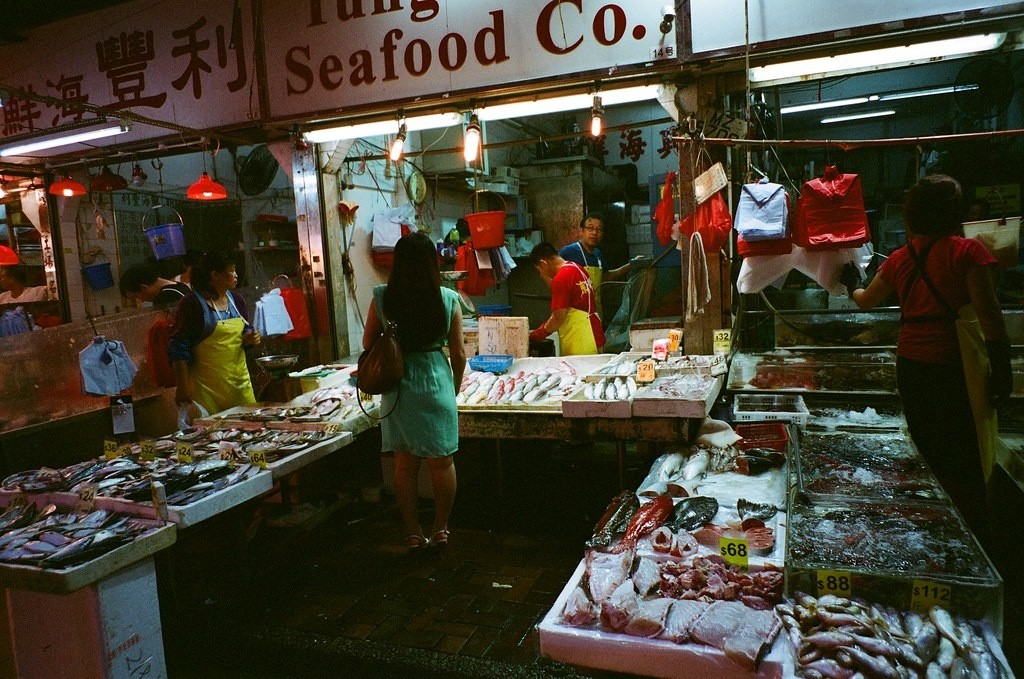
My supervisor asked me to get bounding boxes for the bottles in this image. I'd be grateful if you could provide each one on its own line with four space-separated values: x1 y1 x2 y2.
436 225 472 259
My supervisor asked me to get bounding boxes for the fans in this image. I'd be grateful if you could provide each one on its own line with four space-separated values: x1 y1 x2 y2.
238 146 279 196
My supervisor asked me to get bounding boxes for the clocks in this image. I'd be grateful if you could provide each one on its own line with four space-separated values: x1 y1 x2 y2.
408 171 427 203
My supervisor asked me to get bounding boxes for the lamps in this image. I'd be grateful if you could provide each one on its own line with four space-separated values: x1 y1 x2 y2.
463 99 480 160
0 242 20 264
591 80 604 136
90 161 129 192
390 109 407 161
188 148 226 198
45 168 86 196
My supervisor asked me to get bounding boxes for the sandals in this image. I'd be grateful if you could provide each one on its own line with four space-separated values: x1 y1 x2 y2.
405 531 450 562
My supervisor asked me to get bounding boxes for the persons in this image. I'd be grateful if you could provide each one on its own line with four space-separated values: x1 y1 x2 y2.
361 232 468 557
119 248 261 428
527 242 606 446
838 173 1014 540
541 213 645 323
0 265 48 305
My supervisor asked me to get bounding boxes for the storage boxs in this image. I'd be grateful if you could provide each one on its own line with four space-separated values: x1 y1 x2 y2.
488 166 520 195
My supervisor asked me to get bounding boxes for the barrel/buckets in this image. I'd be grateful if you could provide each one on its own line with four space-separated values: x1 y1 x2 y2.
961 189 1021 269
270 275 312 341
464 189 507 250
477 305 513 315
82 251 114 290
141 203 186 259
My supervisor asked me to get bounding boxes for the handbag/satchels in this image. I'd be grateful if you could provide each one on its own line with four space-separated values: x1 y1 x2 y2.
356 284 403 420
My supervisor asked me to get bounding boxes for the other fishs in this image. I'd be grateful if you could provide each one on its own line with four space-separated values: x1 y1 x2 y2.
49 455 262 508
584 356 649 402
122 423 332 464
554 424 1012 679
215 386 374 425
1 501 141 568
802 319 875 347
457 368 583 403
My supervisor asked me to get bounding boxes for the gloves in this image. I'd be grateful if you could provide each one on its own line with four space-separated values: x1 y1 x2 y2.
527 319 550 343
629 255 654 275
837 260 865 300
986 336 1013 396
